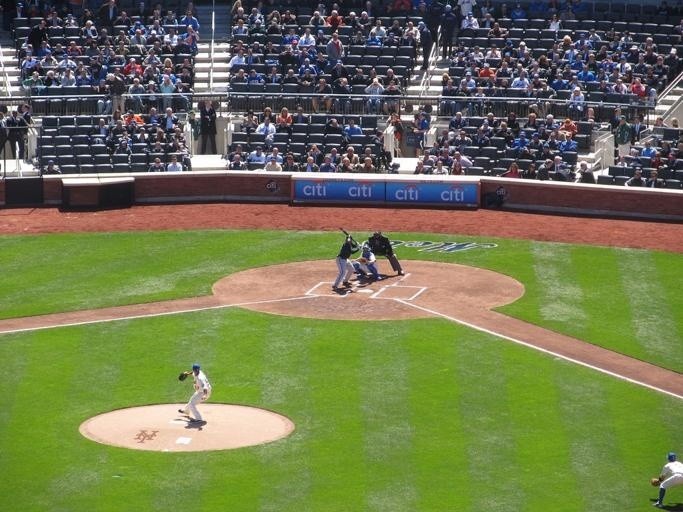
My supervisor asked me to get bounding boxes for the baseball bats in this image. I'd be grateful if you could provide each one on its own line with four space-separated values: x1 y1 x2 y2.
339 226 362 248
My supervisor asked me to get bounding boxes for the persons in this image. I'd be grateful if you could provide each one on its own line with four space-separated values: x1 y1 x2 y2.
368 231 405 275
652 452 683 507
178 364 212 423
332 234 362 291
353 244 382 280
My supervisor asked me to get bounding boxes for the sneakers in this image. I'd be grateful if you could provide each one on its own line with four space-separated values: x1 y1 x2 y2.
178 409 203 425
651 500 664 508
331 281 352 292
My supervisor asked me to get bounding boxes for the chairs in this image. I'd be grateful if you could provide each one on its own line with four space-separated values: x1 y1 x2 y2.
32 112 190 176
418 115 596 179
594 124 683 191
223 111 386 174
226 6 430 111
11 2 196 110
438 9 682 115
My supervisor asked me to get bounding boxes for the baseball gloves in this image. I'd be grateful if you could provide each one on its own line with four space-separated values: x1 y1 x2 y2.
179 372 187 381
651 478 662 486
357 257 367 263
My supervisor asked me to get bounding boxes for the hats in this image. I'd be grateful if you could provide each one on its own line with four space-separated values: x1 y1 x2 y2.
467 11 473 18
192 364 200 370
666 452 676 462
346 234 352 243
0 0 683 177
